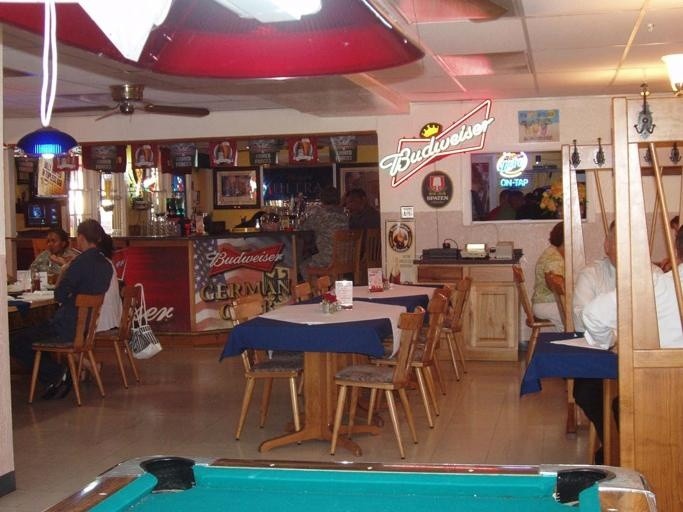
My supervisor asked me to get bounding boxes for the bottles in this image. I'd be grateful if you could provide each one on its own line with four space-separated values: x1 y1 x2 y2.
32 269 39 292
261 198 305 230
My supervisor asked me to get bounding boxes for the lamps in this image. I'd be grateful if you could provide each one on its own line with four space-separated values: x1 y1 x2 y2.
17 2 78 155
661 53 683 97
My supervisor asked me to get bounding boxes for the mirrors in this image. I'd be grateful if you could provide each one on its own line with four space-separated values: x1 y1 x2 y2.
462 143 596 225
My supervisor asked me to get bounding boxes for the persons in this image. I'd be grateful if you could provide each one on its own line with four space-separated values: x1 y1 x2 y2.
471 186 559 219
532 214 683 465
298 184 381 281
12 219 125 402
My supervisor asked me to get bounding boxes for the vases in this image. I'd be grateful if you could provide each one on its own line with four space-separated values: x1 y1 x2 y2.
556 204 585 219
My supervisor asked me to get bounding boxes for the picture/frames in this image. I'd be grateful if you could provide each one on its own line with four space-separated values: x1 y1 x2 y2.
335 161 379 214
213 165 260 210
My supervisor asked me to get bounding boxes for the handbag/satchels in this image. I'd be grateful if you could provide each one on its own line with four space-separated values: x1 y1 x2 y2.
125 325 163 359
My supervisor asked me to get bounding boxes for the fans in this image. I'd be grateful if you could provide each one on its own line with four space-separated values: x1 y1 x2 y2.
51 83 209 122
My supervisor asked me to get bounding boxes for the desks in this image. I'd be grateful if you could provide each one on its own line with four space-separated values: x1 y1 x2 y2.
43 453 658 512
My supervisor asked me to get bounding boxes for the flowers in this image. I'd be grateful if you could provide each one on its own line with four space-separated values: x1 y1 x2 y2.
539 176 586 212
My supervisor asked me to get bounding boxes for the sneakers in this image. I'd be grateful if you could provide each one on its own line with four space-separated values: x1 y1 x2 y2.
39 359 103 399
